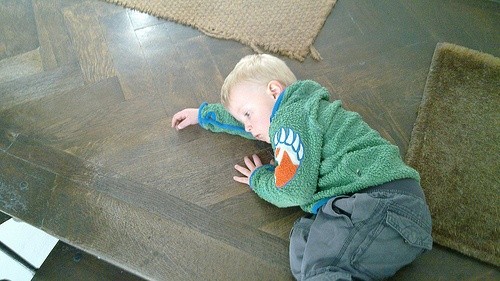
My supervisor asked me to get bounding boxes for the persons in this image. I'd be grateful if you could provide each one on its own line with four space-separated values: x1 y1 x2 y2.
171 53 435 281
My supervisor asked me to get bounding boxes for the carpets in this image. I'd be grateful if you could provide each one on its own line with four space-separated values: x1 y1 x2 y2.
106 0 338 65
404 42 500 268
